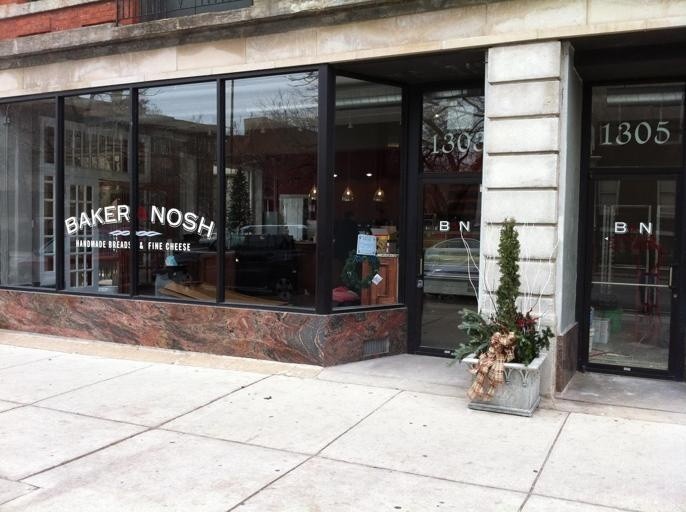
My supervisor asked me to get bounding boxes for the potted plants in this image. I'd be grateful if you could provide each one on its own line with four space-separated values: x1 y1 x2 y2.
448 216 553 418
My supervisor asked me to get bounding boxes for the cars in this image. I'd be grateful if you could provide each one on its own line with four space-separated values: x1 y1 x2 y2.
424 238 480 299
167 224 334 301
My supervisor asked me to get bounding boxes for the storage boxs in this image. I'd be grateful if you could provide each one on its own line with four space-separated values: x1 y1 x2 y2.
588 304 624 352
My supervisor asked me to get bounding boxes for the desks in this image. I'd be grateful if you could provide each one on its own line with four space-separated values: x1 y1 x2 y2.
359 253 398 305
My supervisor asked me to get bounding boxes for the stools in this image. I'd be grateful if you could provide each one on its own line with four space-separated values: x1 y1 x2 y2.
332 286 359 307
